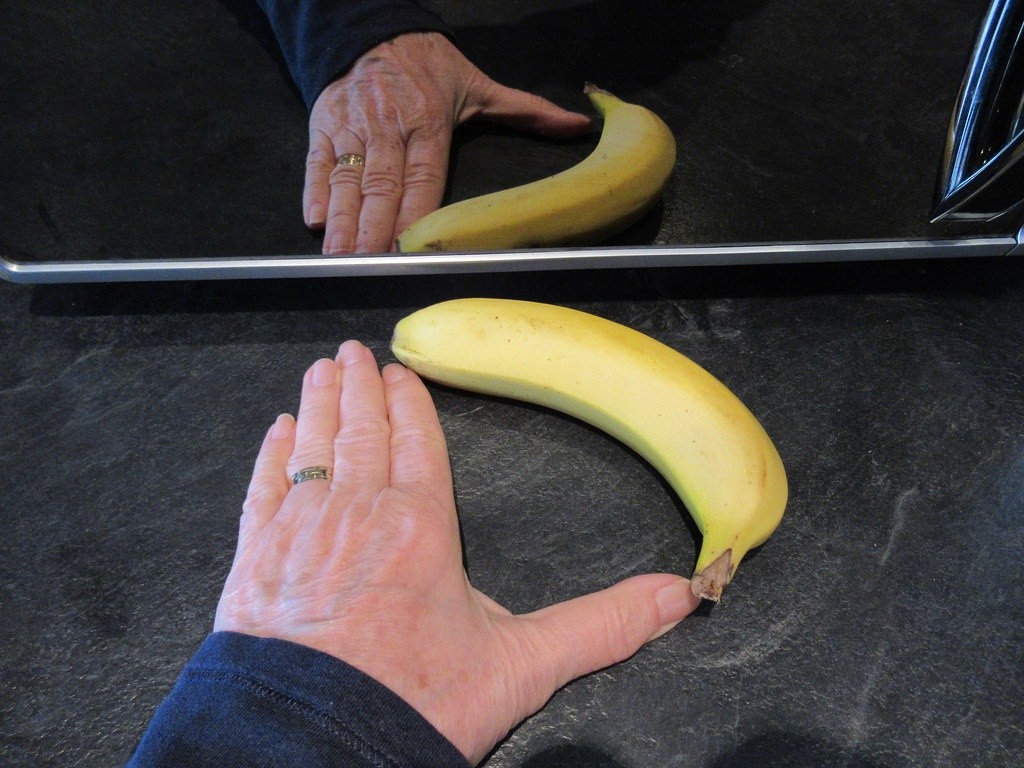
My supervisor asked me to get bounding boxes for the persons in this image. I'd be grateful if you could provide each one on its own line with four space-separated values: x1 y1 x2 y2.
125 338 702 768
256 0 592 254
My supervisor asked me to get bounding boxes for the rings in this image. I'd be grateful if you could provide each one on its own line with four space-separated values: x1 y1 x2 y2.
336 154 365 167
291 466 333 484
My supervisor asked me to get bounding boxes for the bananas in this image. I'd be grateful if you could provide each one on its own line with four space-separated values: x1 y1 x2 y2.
388 297 789 601
392 82 678 251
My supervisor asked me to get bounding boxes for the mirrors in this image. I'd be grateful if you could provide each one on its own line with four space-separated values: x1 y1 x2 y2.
0 1 1024 285
